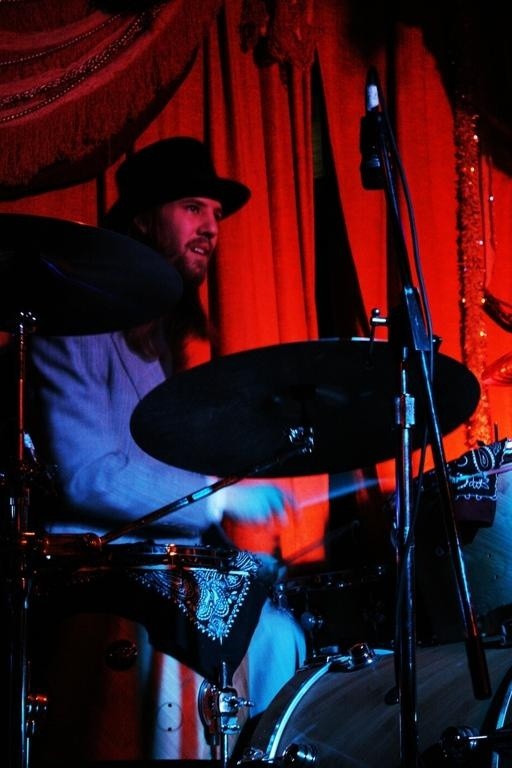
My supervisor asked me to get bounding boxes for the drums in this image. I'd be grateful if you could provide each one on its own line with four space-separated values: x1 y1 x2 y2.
378 438 512 648
2 532 263 768
237 625 512 768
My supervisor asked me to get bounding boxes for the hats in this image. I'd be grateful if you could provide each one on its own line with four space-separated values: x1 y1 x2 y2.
108 136 250 239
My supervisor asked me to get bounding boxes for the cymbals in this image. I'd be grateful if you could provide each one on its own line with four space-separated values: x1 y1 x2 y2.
130 335 480 478
1 213 186 338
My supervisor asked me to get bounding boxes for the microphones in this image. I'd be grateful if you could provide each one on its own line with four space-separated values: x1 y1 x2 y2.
358 68 388 190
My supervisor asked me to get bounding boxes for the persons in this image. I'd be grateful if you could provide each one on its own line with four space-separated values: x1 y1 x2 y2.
0 136 310 765
